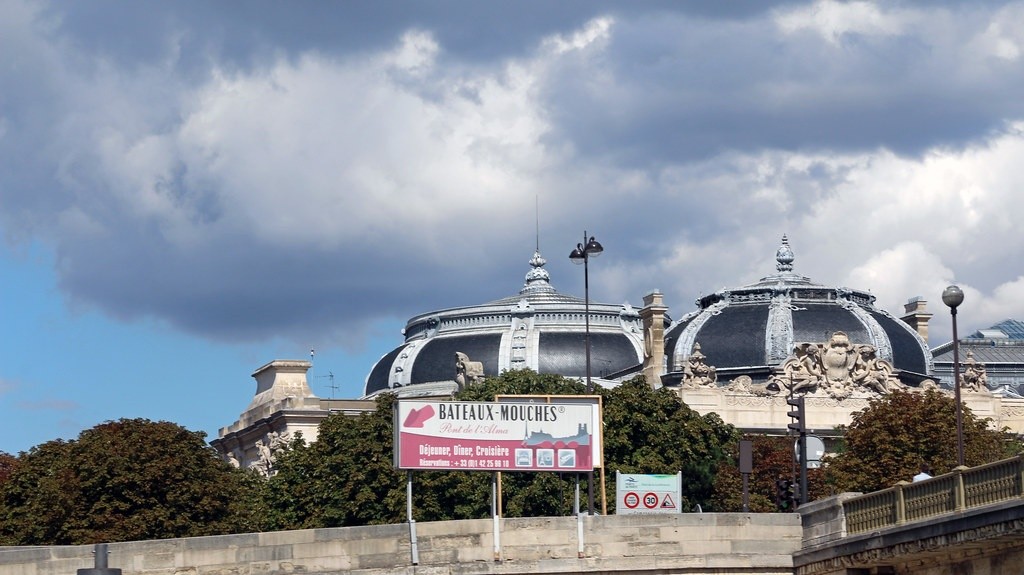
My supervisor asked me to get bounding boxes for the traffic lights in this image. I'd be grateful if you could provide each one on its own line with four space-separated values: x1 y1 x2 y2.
787 397 805 434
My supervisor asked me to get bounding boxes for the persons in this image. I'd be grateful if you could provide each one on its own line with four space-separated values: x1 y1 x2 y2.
973 363 986 388
680 356 699 385
852 347 893 395
699 366 717 387
247 440 270 475
959 374 978 392
227 451 239 468
787 345 823 397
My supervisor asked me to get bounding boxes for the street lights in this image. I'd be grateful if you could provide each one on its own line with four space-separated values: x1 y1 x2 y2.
568 231 605 394
941 285 966 465
